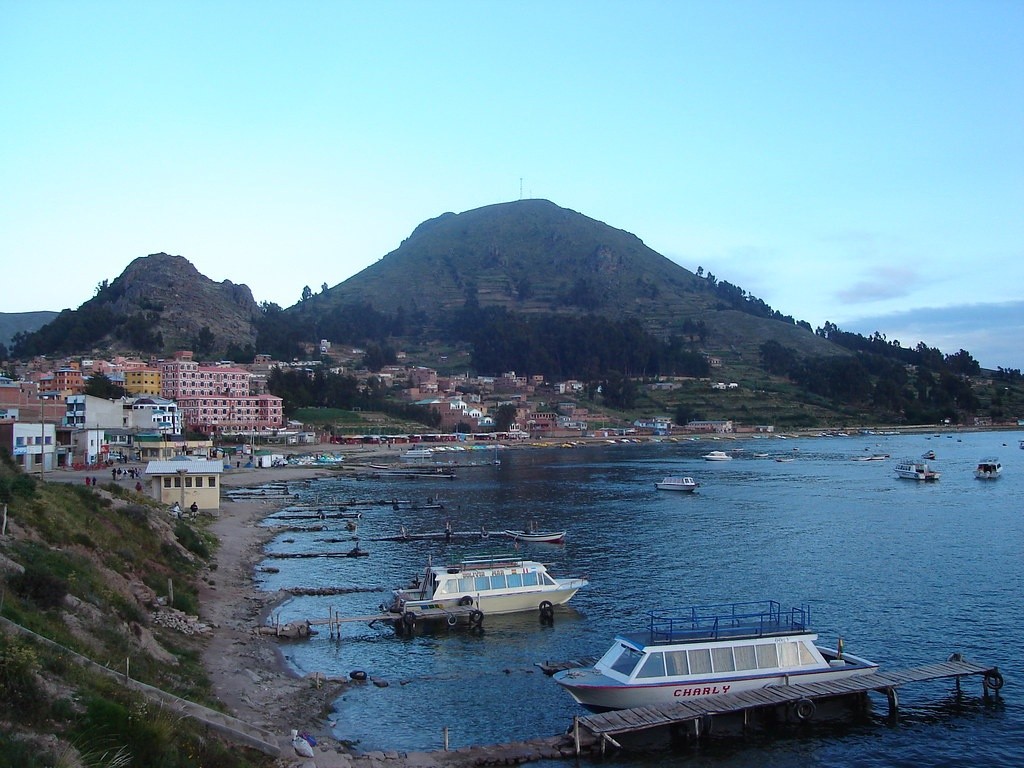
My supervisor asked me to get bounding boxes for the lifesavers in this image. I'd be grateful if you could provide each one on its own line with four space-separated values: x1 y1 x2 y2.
470 610 484 623
263 470 458 522
946 652 958 662
794 698 816 721
458 596 473 606
539 600 553 612
654 484 658 488
984 671 1003 690
447 569 460 574
445 614 458 625
481 530 490 538
403 612 416 625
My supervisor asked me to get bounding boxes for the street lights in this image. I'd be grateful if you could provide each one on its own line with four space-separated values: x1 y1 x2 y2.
164 425 168 461
251 428 255 467
40 394 50 480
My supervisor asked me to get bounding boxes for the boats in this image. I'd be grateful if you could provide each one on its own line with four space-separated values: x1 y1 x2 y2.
653 473 700 492
893 457 942 481
851 452 890 462
388 551 592 620
973 456 1004 479
434 430 903 454
776 459 794 462
399 443 433 458
921 448 936 459
552 597 880 714
753 452 769 457
701 450 733 462
504 521 567 543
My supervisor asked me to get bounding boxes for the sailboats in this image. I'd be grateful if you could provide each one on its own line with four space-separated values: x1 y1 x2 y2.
492 443 501 467
